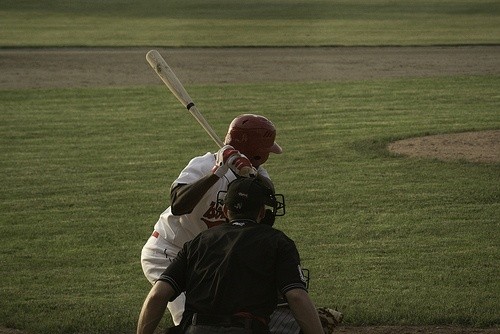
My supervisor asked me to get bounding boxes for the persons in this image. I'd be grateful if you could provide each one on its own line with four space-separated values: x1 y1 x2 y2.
141 113 283 326
136 173 325 334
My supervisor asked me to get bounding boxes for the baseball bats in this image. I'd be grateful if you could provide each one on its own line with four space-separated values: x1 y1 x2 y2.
145 50 250 176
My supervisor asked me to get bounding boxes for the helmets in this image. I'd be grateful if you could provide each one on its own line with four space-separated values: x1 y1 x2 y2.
225 175 278 227
225 114 282 154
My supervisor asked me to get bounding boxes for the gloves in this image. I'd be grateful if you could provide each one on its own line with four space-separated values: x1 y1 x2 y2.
212 145 241 178
228 154 257 179
